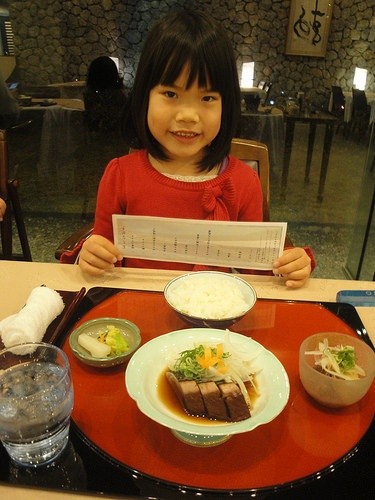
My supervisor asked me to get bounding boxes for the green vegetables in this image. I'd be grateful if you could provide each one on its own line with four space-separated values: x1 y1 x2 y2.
97 325 129 356
331 347 355 372
172 344 231 383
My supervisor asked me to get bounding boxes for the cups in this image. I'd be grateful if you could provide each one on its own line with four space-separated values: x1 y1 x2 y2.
0 341 74 467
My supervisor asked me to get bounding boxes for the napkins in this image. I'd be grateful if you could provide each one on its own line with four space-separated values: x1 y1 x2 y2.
0 287 64 355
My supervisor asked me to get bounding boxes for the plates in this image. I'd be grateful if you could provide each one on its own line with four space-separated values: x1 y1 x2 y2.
124 327 291 436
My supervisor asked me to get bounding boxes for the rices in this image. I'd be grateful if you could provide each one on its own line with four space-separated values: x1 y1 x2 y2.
167 273 250 318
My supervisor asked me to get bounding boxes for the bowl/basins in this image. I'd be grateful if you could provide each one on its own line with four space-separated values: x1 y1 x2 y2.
298 331 375 410
18 97 32 107
69 316 141 369
163 270 257 330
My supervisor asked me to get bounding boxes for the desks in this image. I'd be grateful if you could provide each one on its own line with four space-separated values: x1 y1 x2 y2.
328 92 375 142
16 98 86 175
275 98 337 202
237 103 283 183
47 81 86 98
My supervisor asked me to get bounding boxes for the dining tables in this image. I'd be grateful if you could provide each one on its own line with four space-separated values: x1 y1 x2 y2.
0 258 375 500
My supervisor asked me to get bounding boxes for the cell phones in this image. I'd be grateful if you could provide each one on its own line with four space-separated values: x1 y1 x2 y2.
336 290 375 306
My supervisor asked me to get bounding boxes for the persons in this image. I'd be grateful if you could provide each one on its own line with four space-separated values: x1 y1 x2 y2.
61 10 316 288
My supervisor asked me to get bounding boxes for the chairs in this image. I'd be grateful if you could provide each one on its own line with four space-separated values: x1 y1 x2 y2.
0 129 31 262
55 139 295 261
330 85 347 136
347 87 371 144
